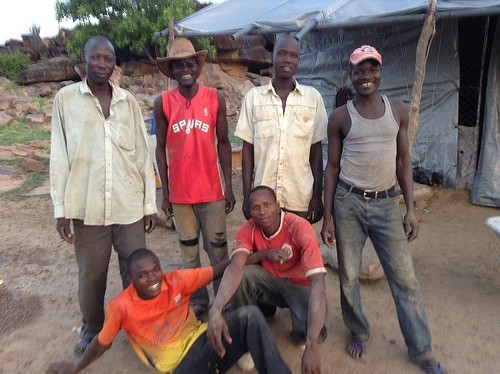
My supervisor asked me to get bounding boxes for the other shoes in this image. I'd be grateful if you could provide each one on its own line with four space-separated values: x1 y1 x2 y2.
294 338 306 348
237 351 256 371
191 303 208 319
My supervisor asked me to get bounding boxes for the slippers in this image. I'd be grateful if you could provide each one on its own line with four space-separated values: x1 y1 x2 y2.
73 337 94 357
415 358 449 374
346 337 371 361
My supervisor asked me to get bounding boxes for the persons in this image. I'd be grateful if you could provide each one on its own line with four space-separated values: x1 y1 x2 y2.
206 185 328 374
50 34 159 356
153 37 235 318
318 45 446 374
233 34 330 319
46 248 292 374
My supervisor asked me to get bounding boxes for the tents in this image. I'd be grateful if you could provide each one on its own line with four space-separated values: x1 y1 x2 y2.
150 0 500 209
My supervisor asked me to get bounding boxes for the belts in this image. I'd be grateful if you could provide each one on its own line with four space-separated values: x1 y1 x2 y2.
338 179 404 200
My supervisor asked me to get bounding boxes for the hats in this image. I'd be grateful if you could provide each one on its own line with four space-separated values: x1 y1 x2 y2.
156 37 208 80
348 45 383 69
